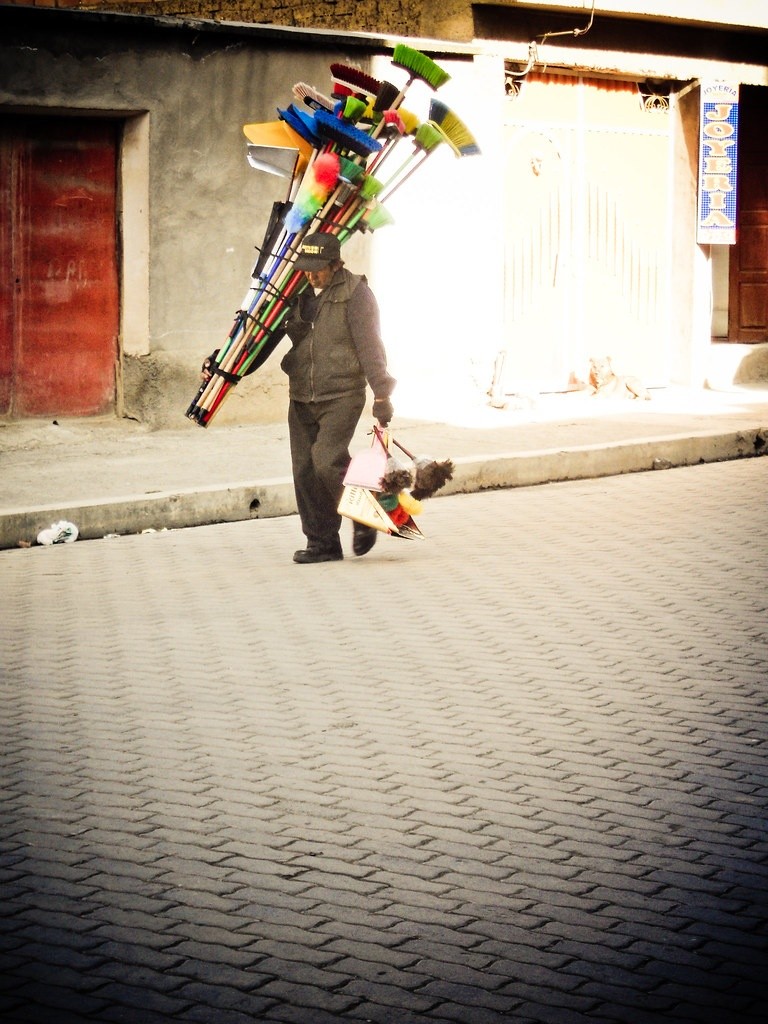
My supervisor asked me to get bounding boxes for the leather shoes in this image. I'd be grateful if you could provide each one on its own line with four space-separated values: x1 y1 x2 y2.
292 543 344 563
353 520 377 556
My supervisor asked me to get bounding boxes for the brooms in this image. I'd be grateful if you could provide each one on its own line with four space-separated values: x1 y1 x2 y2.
184 41 484 431
371 426 455 501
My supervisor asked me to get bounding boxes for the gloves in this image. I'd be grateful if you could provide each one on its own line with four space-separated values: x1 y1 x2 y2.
373 398 395 428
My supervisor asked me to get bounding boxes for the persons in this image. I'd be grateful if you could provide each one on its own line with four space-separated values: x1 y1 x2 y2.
200 231 398 564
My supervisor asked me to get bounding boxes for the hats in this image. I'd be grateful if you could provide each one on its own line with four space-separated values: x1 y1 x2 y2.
293 233 341 271
